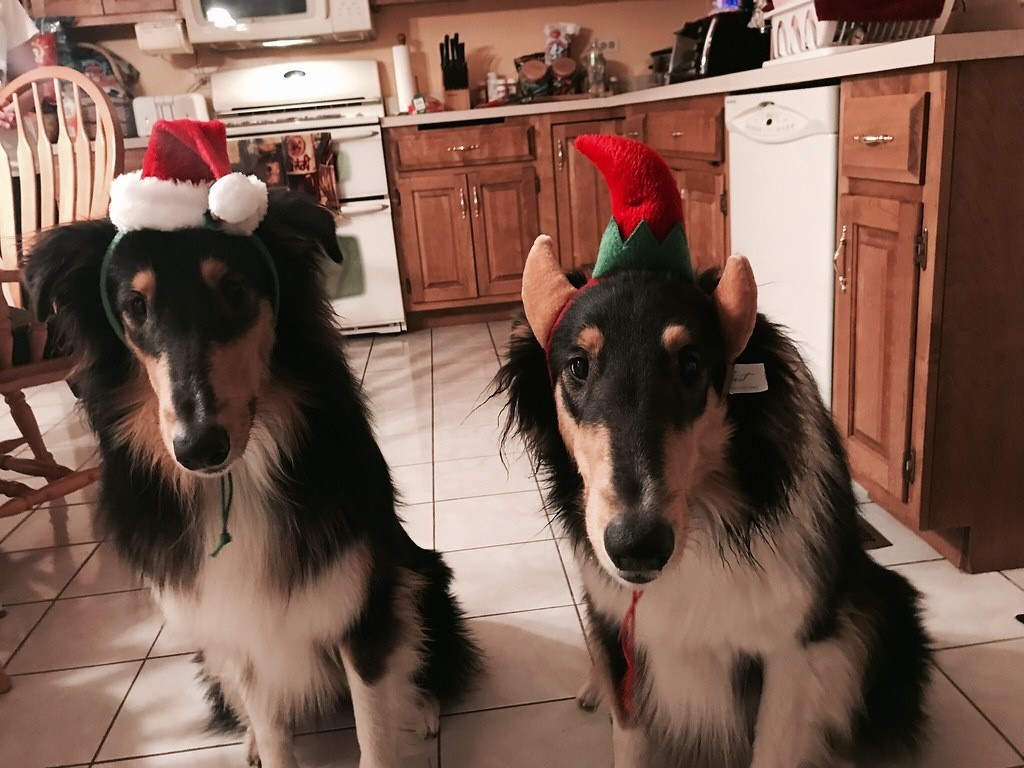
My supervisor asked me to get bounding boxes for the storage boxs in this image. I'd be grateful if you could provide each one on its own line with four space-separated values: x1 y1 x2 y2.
764 0 850 66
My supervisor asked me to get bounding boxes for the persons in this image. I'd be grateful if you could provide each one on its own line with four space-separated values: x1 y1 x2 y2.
2 1 68 367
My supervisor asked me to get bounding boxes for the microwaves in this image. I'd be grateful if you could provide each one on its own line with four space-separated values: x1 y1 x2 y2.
662 0 771 82
182 0 377 48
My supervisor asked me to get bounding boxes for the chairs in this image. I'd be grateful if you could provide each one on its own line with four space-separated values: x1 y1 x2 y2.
0 62 127 517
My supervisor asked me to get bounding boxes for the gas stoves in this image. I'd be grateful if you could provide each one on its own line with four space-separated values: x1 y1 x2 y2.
213 113 380 136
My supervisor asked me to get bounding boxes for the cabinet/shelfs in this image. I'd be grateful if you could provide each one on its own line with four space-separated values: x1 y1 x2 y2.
832 65 1024 577
535 105 622 281
625 94 727 283
380 113 544 311
27 1 188 26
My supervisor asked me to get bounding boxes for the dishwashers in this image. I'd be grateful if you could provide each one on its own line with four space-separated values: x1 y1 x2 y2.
723 86 835 414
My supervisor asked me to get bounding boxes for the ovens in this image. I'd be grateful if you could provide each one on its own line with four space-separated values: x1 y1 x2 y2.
212 125 408 334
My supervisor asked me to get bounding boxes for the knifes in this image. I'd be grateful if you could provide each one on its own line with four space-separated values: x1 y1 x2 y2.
439 33 465 67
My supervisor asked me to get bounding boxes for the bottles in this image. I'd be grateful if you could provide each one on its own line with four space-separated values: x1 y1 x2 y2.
496 80 507 98
487 73 499 102
586 40 607 95
507 78 517 95
478 82 488 103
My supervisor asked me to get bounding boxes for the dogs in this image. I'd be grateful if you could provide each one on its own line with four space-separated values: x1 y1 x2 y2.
10 189 494 768
458 261 940 766
